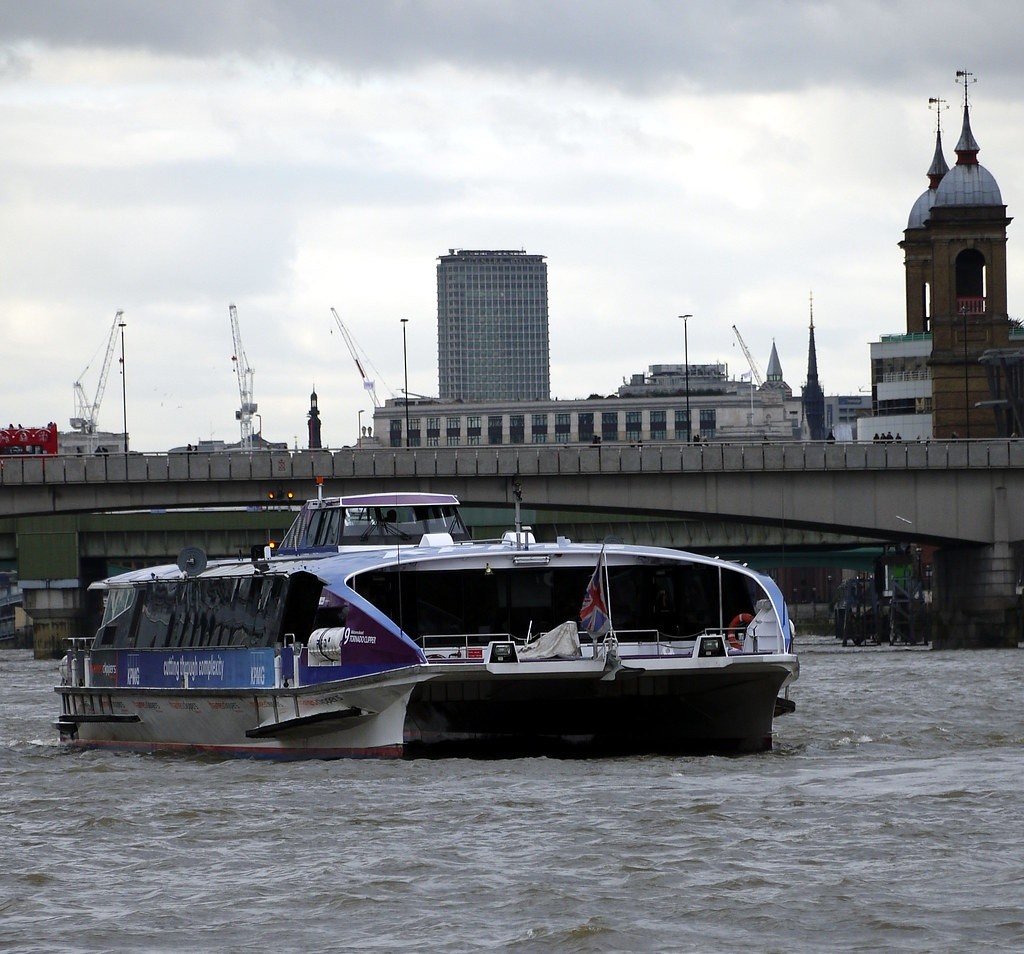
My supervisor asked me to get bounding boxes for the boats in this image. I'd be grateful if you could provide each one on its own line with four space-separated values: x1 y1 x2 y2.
50 481 799 762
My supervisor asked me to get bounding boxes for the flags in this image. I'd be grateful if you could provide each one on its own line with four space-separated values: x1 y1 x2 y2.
364 381 375 389
579 545 611 641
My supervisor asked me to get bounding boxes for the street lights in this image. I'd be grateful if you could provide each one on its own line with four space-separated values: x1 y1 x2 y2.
358 410 364 448
400 319 410 451
960 306 971 437
679 315 693 447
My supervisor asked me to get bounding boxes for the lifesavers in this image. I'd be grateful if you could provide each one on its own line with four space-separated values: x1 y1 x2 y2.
726 612 755 650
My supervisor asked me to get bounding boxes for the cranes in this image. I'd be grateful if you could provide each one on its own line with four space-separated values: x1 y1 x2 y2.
732 325 763 388
330 307 395 408
69 311 123 457
229 305 257 454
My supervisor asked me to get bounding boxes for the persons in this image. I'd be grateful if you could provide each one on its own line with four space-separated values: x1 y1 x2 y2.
693 434 708 447
593 435 601 448
637 438 643 447
951 431 958 443
873 431 902 444
926 436 930 446
630 439 635 448
917 436 920 444
826 432 835 444
762 436 769 446
